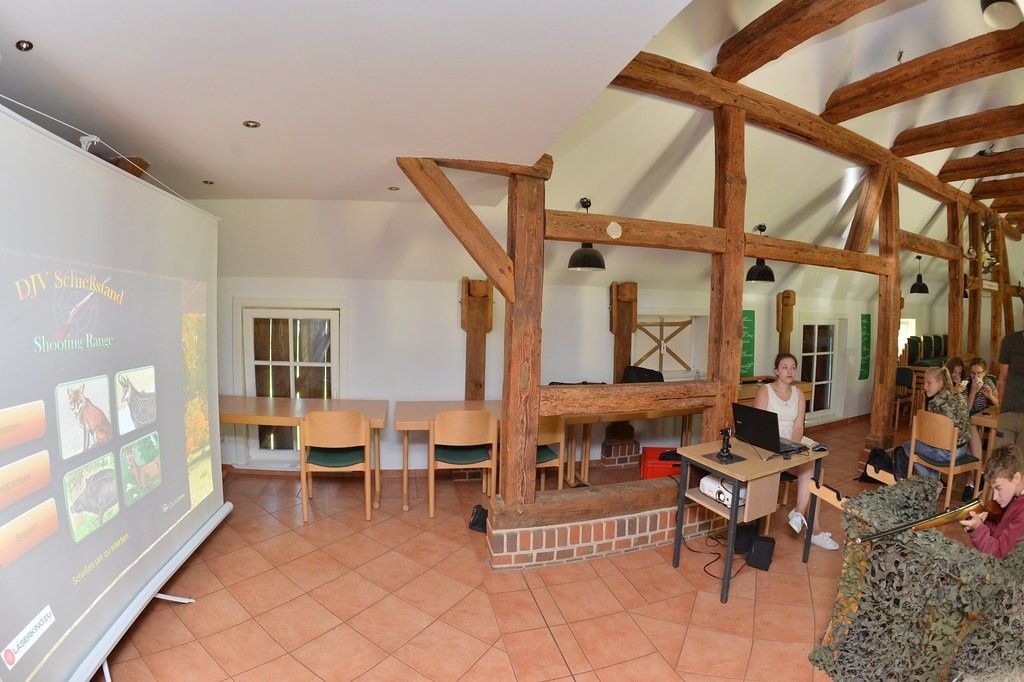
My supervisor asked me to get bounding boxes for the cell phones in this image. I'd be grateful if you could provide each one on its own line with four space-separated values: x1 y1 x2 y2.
960 381 969 387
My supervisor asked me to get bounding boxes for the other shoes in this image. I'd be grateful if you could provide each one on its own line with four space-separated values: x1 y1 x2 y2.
961 484 975 503
940 478 947 489
979 472 985 492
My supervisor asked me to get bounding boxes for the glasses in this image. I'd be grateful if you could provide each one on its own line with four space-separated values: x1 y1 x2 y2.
969 370 985 376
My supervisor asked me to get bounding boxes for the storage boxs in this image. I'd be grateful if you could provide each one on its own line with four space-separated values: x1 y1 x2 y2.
641 447 683 480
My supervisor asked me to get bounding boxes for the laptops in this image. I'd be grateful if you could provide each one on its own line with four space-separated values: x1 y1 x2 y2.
732 402 806 455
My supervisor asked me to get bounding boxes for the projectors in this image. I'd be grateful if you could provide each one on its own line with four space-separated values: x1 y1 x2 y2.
700 474 747 508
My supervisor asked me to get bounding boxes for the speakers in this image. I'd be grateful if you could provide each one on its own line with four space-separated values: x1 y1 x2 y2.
746 536 776 572
736 525 756 554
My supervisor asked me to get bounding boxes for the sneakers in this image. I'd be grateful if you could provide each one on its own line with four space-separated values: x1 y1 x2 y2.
788 507 808 535
804 531 840 551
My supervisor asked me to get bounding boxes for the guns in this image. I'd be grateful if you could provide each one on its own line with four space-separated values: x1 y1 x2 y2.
854 496 1016 545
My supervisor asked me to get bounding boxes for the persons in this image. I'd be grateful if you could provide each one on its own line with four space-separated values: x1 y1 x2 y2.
958 443 1024 558
754 353 839 550
903 356 999 502
994 310 1024 456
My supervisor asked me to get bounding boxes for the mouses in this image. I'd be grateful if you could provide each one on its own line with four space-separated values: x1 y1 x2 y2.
812 444 827 451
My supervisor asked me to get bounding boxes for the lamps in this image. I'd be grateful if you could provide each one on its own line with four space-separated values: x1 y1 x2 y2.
910 256 929 295
567 200 607 272
964 290 968 298
745 225 775 281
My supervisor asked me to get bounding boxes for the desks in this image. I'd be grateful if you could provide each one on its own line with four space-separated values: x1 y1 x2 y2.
393 400 599 511
971 404 1000 462
219 395 389 509
575 406 704 488
673 438 829 603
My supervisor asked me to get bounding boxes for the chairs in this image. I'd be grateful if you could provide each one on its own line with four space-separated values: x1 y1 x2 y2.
536 415 565 490
299 412 371 521
765 468 824 536
428 411 495 518
895 335 997 513
